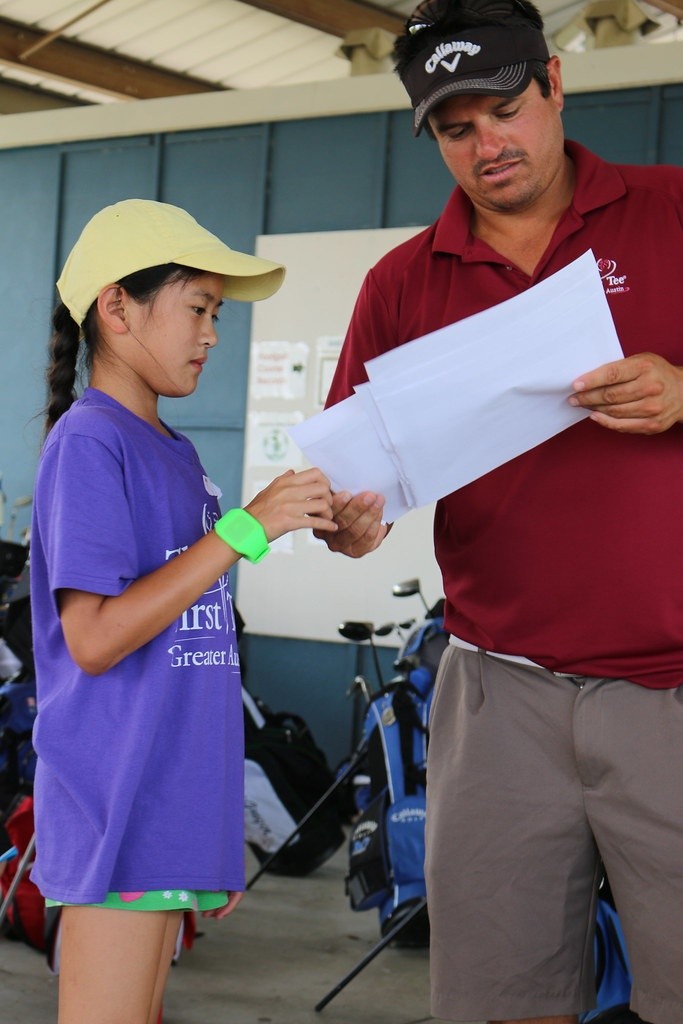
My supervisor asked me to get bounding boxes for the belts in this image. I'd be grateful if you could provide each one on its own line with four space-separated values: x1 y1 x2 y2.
448 632 583 678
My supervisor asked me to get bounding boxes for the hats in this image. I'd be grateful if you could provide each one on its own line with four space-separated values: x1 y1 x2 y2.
399 23 550 138
55 200 285 325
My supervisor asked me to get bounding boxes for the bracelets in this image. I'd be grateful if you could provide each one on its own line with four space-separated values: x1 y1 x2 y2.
213 508 271 564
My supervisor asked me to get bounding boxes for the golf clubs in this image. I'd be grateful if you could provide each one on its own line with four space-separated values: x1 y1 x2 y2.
313 899 428 1014
336 577 436 704
244 752 364 892
0 830 36 926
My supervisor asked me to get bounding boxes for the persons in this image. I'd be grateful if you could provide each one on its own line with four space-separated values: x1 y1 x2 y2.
29 199 338 1024
308 0 683 1024
